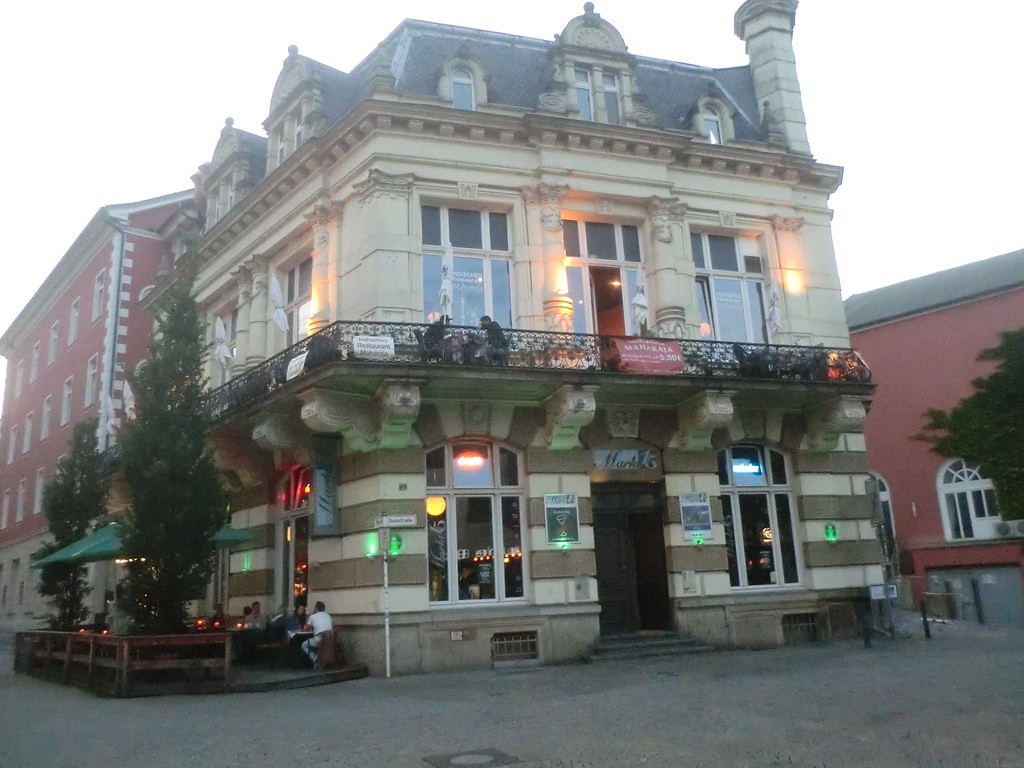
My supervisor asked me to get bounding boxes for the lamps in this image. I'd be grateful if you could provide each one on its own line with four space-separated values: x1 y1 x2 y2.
427 496 446 515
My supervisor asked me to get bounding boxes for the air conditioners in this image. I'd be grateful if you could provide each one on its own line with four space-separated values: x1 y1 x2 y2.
989 519 1024 538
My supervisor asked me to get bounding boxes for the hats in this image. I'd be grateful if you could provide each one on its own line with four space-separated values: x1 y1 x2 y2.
440 315 453 320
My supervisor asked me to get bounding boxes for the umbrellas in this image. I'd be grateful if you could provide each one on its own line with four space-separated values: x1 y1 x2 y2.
214 316 233 369
439 240 453 324
270 272 290 333
31 522 266 634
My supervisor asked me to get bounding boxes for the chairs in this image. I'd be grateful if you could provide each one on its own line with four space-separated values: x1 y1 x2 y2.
732 343 763 378
496 332 513 365
414 329 443 363
302 628 339 670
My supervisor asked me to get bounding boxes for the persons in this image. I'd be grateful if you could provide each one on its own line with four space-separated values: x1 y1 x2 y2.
477 316 508 362
211 603 224 621
236 601 266 629
439 559 479 600
424 313 453 361
286 603 304 631
302 601 333 669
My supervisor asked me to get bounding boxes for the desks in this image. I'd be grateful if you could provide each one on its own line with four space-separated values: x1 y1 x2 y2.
288 631 313 640
445 333 489 363
